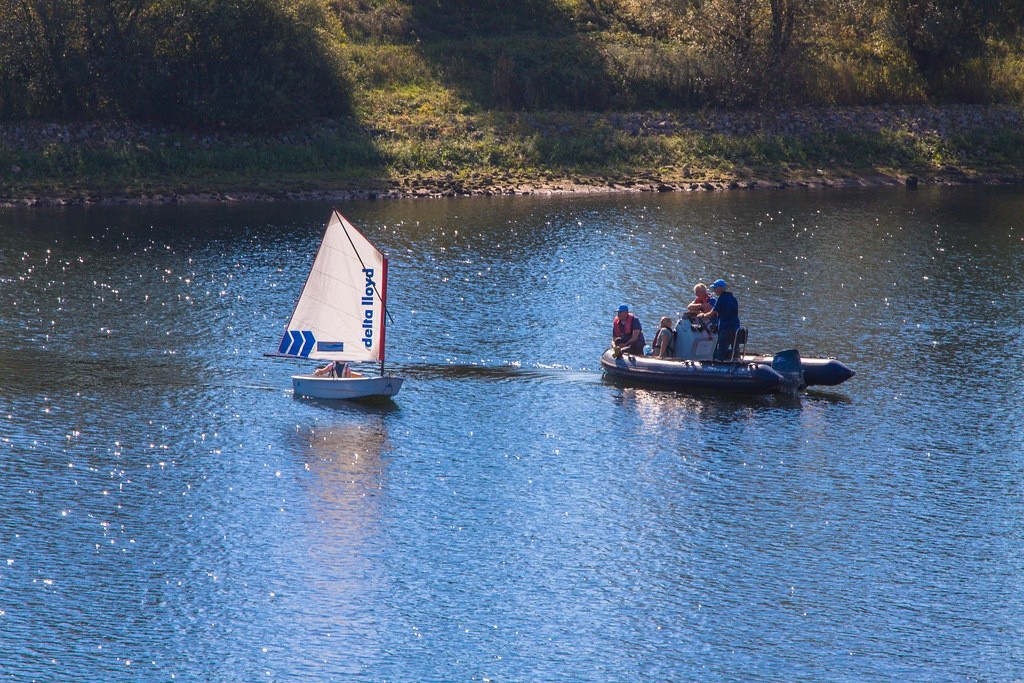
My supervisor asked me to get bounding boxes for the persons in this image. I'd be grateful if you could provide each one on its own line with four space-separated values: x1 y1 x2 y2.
688 283 720 329
643 316 674 359
611 304 646 356
314 361 352 378
697 279 740 361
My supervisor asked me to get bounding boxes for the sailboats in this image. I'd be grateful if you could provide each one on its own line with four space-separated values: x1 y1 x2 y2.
260 208 406 402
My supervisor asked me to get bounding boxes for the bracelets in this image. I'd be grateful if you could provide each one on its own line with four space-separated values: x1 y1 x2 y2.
702 315 704 318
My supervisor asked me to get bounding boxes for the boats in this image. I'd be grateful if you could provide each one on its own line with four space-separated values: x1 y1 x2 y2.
599 348 856 387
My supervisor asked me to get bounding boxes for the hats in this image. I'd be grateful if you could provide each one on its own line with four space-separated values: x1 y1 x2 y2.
710 279 727 288
615 304 628 312
